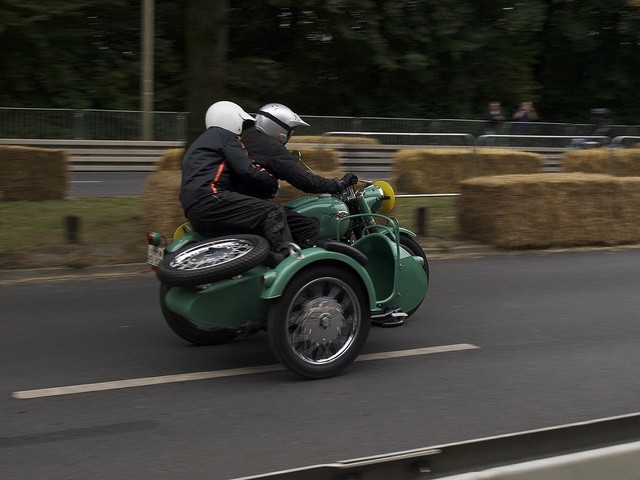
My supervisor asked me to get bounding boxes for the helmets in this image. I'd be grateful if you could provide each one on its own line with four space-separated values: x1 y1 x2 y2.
255 103 310 144
205 101 257 135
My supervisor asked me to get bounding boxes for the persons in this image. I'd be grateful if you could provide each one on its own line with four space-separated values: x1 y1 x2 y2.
240 103 358 248
180 100 295 259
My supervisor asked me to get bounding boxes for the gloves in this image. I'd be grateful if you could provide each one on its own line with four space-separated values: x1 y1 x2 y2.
334 173 358 194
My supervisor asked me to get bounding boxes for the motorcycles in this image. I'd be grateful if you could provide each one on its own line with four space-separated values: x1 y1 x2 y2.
146 150 429 380
